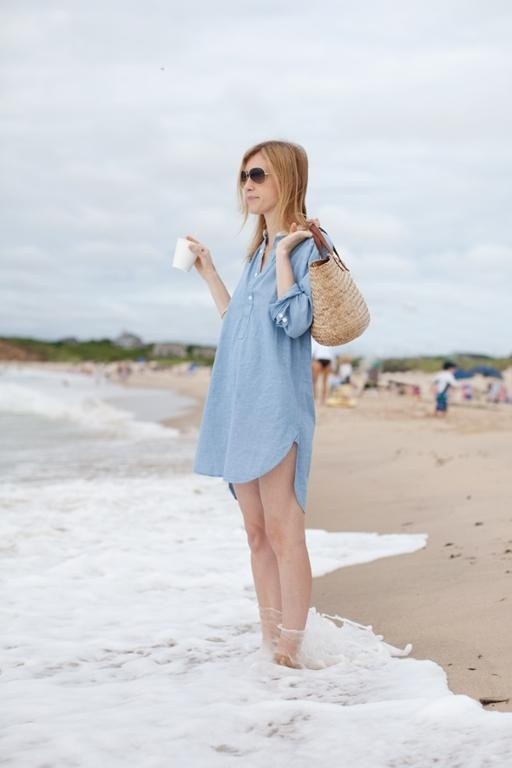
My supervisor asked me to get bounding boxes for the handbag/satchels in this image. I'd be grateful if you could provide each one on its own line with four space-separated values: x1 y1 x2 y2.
308 255 371 346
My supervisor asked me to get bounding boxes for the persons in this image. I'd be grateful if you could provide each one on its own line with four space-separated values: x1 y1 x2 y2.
186 141 333 665
310 335 338 405
430 361 460 415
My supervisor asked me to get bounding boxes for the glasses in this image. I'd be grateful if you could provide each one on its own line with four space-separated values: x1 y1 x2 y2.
240 168 275 186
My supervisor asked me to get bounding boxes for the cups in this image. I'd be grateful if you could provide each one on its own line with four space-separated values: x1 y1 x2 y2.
172 238 200 273
